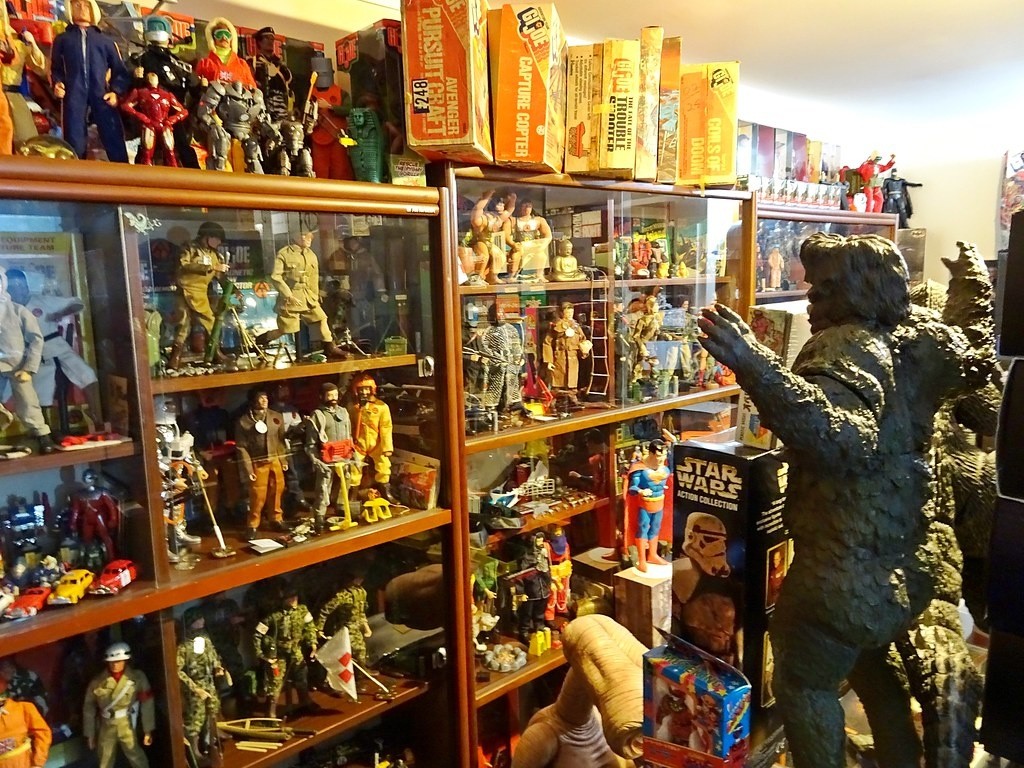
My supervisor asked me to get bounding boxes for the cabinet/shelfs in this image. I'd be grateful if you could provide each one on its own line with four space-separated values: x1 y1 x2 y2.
0 156 468 768
756 201 899 310
444 165 761 766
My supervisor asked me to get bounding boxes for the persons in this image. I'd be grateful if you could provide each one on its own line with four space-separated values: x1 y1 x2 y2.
70 468 123 560
628 439 669 574
768 552 785 607
835 150 924 230
569 428 611 496
169 221 388 373
468 189 588 285
615 294 725 405
0 0 353 182
670 511 738 671
177 565 372 768
697 229 997 768
461 301 590 436
0 643 156 767
470 526 574 648
0 269 99 455
152 375 401 563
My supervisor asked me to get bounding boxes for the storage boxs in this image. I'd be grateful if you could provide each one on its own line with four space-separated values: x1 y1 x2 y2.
642 294 814 768
400 1 844 211
